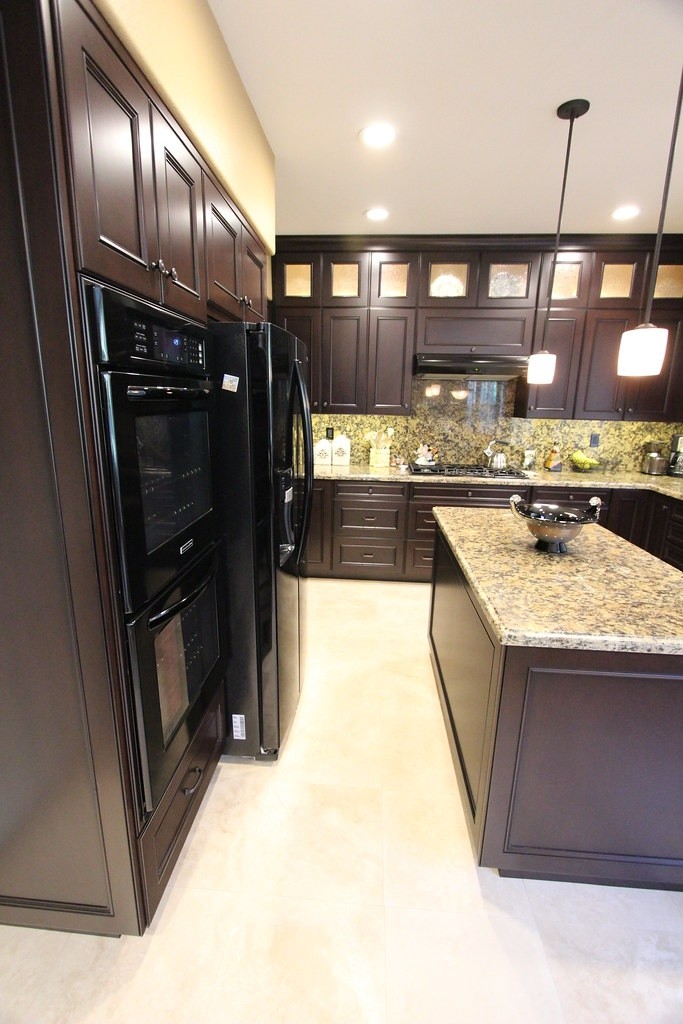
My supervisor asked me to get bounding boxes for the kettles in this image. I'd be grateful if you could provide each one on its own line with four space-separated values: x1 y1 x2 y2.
483 439 509 470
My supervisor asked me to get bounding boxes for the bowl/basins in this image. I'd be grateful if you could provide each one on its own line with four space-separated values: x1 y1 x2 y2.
509 494 602 554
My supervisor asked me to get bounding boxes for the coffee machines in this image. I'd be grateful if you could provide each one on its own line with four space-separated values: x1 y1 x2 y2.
667 433 683 478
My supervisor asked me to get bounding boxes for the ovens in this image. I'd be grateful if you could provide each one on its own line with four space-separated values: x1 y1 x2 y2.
85 282 230 816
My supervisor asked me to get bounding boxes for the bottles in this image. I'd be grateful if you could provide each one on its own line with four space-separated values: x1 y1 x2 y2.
548 441 562 472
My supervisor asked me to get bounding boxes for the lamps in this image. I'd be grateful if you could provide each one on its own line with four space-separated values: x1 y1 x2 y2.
527 99 590 383
618 70 683 376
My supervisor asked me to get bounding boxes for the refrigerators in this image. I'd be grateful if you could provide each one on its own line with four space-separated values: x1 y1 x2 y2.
206 321 314 761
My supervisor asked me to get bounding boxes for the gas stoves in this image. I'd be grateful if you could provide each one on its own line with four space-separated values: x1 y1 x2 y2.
408 462 530 479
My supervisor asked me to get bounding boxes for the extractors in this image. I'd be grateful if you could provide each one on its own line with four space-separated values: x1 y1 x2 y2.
412 353 529 382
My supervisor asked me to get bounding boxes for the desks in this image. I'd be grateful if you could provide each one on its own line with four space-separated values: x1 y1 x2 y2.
427 505 683 894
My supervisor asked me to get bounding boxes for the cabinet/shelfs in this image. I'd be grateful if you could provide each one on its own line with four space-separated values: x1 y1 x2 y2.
269 251 683 422
0 0 271 937
306 480 683 584
